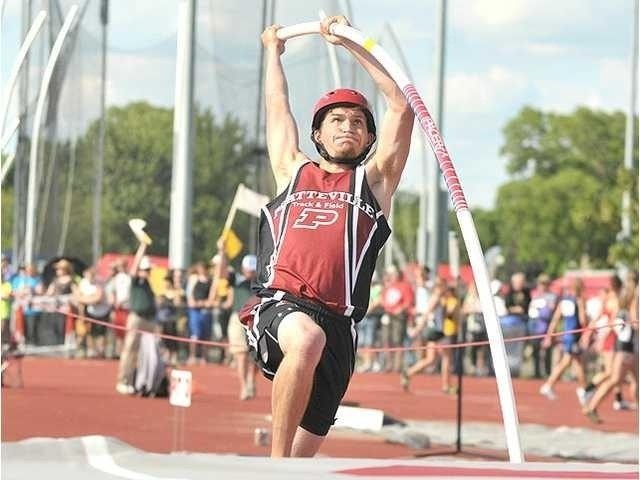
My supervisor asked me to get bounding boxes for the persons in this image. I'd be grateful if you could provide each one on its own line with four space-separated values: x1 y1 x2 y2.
356 265 640 423
46 240 257 401
0 256 79 344
239 13 415 460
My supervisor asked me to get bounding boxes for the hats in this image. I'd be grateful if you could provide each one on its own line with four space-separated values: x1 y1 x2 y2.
242 254 256 272
138 255 151 270
53 259 74 274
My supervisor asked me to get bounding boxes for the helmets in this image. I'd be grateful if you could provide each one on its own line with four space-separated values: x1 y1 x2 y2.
310 88 375 141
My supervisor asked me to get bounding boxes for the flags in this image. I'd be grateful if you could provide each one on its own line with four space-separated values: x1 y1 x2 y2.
236 185 270 219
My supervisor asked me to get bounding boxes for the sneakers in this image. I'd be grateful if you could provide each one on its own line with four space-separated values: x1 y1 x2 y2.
442 386 458 394
240 389 251 400
400 370 409 392
248 384 255 398
539 383 556 401
582 405 602 423
116 383 127 394
576 386 588 405
613 400 634 410
127 384 135 395
